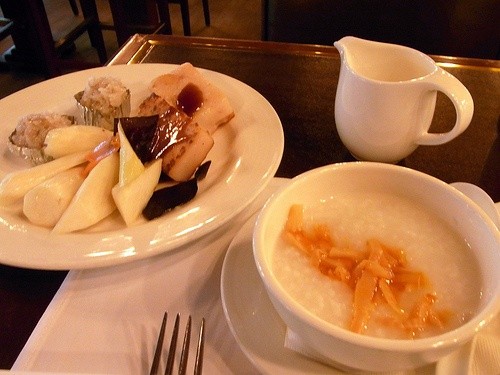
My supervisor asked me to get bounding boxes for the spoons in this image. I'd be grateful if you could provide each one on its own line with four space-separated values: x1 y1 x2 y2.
432 176 498 374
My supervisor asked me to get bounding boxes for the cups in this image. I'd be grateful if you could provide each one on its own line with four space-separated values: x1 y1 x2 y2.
330 35 474 164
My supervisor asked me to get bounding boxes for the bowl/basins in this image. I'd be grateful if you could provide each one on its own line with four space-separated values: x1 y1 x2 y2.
251 161 499 372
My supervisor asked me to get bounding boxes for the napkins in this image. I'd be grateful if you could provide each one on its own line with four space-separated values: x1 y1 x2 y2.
9 177 500 375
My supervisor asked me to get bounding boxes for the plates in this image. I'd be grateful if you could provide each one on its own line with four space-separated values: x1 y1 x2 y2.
0 62 284 271
218 205 433 374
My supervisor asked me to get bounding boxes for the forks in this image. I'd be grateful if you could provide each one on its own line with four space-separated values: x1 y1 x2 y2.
143 307 204 374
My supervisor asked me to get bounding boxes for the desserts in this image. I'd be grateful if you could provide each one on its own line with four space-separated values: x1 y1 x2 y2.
7 74 132 161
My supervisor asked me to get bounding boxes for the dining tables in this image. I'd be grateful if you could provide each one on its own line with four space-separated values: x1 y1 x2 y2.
0 32 499 375
